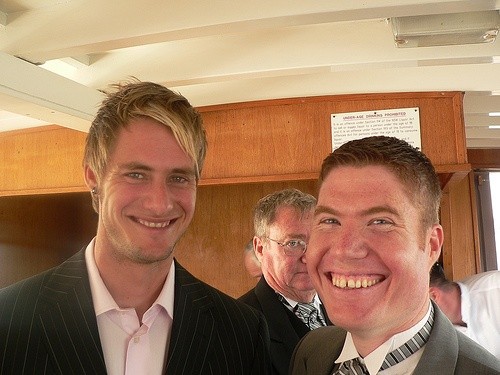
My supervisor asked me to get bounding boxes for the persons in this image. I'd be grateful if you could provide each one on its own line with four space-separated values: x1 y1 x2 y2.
288 135 500 375
0 75 269 375
243 238 262 282
236 188 334 375
429 261 500 360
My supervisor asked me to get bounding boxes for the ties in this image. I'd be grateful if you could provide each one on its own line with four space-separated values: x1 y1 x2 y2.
275 293 327 330
333 306 434 375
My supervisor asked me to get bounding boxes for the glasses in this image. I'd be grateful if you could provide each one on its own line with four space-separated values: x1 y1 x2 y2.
259 236 306 256
